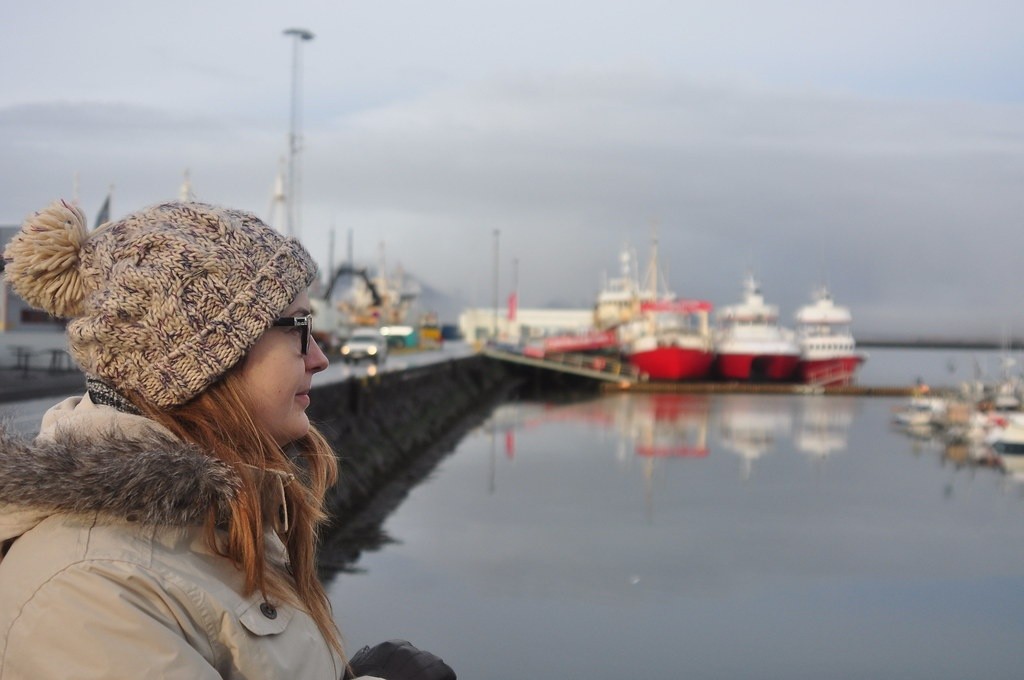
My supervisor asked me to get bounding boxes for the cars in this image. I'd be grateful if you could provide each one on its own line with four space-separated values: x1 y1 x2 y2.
339 326 390 366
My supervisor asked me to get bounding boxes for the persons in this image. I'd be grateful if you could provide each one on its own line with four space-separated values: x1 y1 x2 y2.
0 198 456 680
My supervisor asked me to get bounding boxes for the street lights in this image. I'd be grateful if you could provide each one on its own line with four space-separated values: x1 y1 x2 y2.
278 25 314 238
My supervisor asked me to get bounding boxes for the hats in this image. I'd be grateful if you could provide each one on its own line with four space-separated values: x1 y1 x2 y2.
5 201 318 407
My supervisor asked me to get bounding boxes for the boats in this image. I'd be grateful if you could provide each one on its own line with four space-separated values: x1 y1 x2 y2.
613 389 854 477
593 225 866 387
898 338 1024 461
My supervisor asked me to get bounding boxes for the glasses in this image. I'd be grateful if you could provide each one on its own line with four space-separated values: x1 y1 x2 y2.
276 314 312 356
